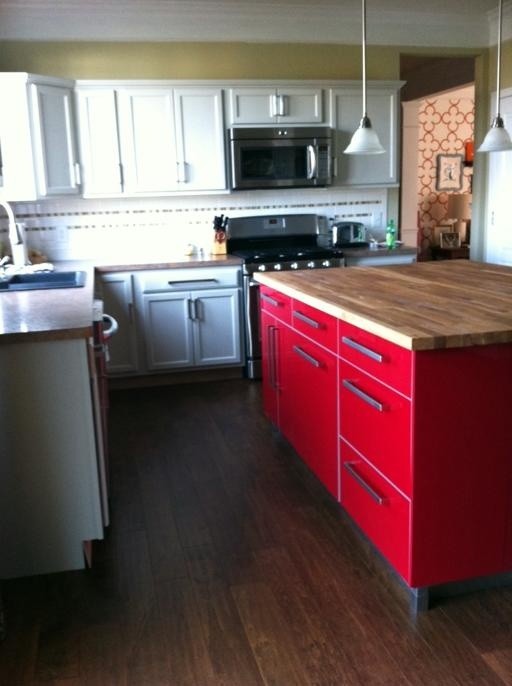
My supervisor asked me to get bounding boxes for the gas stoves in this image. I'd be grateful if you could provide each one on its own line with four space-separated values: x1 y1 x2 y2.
225 213 346 275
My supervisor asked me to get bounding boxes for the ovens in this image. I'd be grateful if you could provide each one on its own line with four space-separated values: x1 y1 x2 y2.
242 275 263 379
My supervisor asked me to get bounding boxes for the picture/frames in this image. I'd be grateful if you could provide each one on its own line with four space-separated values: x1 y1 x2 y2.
440 231 461 249
433 224 452 246
435 154 463 191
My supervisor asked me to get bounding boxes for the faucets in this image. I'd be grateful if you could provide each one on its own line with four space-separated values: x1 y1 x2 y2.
0 200 23 247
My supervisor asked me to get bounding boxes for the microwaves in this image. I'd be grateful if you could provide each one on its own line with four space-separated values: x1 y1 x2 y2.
223 125 336 192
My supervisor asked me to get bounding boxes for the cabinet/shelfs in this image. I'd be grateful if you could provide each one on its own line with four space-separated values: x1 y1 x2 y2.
260 296 291 430
229 86 277 124
126 86 178 193
31 84 82 197
75 88 123 196
98 273 138 374
133 271 240 376
329 87 397 185
271 313 338 498
90 300 111 528
277 87 322 124
339 324 413 589
173 87 226 191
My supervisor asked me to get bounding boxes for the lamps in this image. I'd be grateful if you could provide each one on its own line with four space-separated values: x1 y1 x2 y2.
343 1 386 156
447 194 472 243
477 1 512 153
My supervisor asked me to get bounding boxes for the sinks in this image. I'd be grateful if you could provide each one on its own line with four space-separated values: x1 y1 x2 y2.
0 270 86 292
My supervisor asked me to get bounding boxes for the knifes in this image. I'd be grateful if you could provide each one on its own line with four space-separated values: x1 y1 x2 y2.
212 214 229 255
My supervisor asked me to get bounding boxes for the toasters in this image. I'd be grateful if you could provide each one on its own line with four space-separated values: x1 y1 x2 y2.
331 221 369 250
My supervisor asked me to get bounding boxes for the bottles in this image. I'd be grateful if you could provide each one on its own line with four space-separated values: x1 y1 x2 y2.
385 218 396 248
